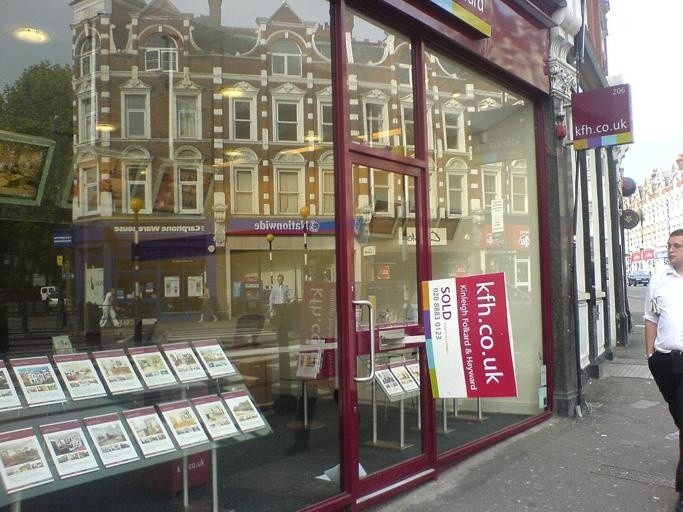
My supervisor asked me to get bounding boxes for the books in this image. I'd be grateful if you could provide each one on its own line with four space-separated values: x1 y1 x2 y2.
123 408 176 457
0 360 23 411
129 345 177 388
192 339 236 378
92 350 143 395
11 356 67 407
159 400 210 449
53 352 107 400
294 338 324 379
1 427 55 493
222 390 265 432
40 420 99 479
83 414 140 468
374 359 420 396
163 342 208 383
193 396 240 440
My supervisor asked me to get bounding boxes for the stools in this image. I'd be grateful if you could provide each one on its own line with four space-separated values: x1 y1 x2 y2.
654 348 683 357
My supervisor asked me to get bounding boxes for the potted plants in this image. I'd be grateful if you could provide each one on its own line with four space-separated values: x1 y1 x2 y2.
366 199 402 240
0 129 219 220
435 208 465 246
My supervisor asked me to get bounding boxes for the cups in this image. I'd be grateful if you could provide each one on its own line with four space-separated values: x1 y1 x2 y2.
666 244 683 249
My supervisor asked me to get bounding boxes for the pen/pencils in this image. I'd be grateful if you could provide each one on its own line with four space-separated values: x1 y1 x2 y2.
40 286 58 303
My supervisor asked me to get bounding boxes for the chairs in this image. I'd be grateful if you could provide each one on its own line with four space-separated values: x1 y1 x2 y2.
677 495 683 511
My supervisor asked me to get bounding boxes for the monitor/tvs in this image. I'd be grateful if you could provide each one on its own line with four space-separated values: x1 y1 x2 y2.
294 338 324 379
374 359 420 396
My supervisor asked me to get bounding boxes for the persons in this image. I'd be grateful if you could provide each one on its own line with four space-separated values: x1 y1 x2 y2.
97 286 121 328
267 274 296 319
642 229 683 511
196 282 218 323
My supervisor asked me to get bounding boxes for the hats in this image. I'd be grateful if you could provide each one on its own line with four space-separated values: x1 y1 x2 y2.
626 271 651 286
46 292 67 310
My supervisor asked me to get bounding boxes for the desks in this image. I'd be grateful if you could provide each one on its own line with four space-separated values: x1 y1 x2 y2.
297 205 311 280
128 193 145 343
267 233 273 289
